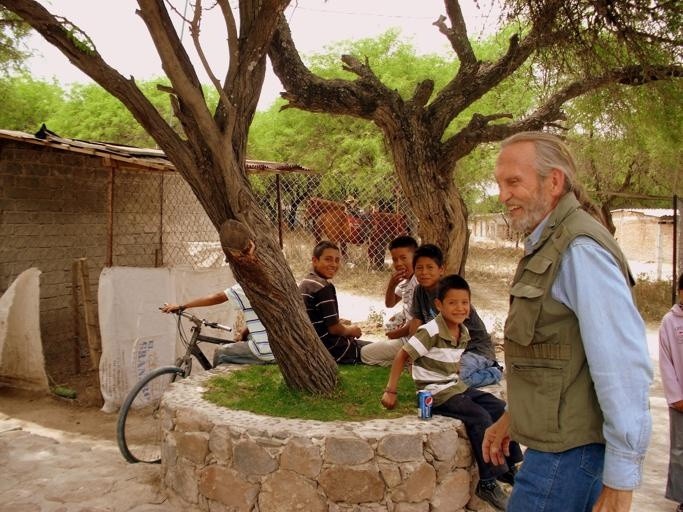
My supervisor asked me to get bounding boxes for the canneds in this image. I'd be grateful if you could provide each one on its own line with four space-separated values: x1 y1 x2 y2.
417 390 433 419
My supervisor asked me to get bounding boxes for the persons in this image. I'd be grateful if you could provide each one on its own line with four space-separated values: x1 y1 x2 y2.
300 240 373 365
480 130 653 512
658 273 683 511
403 243 503 389
380 274 523 512
161 255 277 368
359 235 419 367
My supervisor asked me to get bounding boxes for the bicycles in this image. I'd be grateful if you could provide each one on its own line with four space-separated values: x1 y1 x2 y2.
116 301 247 462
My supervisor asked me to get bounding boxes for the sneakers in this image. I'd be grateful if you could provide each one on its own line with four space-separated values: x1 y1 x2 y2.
497 463 519 487
474 477 507 510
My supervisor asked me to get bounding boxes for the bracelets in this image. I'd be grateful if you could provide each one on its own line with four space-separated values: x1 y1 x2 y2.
179 304 185 311
385 390 398 395
